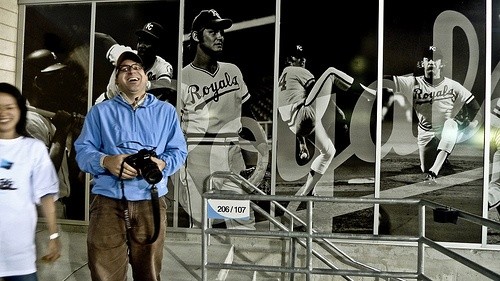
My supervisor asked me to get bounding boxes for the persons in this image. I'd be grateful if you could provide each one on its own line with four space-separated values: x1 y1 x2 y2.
278 44 377 197
180 9 272 230
74 51 189 281
95 21 173 104
383 47 480 186
0 82 62 281
23 49 82 219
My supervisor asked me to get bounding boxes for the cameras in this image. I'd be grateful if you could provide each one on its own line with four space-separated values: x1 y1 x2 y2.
124 148 163 184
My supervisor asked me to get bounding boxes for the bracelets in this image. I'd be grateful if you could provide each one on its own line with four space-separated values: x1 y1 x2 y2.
48 231 60 241
100 155 108 168
